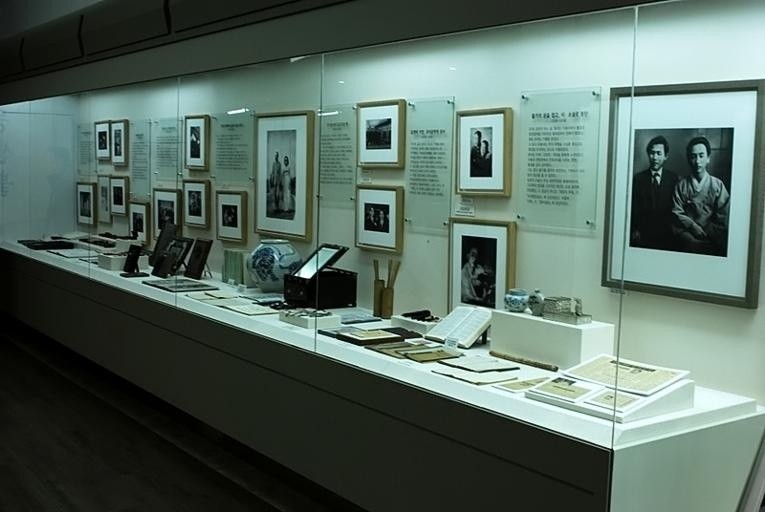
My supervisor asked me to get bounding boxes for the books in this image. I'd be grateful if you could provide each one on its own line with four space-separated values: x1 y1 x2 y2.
221 247 257 289
47 231 150 272
317 312 406 348
424 304 493 349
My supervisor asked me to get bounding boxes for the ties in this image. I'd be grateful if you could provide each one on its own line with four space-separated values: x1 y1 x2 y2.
653 173 659 204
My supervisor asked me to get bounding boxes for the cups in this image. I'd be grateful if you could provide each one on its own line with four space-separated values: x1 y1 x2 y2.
373 278 385 317
382 287 393 318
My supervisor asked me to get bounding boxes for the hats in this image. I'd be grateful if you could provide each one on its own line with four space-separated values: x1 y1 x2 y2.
467 249 478 258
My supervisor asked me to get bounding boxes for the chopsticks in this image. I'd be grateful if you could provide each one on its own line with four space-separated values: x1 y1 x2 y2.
373 258 381 280
387 258 401 288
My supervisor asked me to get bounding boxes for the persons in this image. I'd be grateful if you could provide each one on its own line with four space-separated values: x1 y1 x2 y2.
472 131 491 162
367 207 379 229
631 136 683 250
377 208 388 232
190 192 201 214
269 151 282 210
161 209 171 230
663 136 728 256
367 126 391 146
281 156 292 213
474 141 492 170
461 248 479 303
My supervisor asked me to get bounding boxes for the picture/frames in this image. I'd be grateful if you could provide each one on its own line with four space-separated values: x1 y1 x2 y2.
600 79 765 310
448 217 517 310
74 113 248 246
356 98 406 170
253 110 315 243
454 106 513 197
354 184 404 256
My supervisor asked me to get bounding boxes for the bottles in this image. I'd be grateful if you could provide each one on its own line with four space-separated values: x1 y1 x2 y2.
527 287 544 316
504 289 530 313
